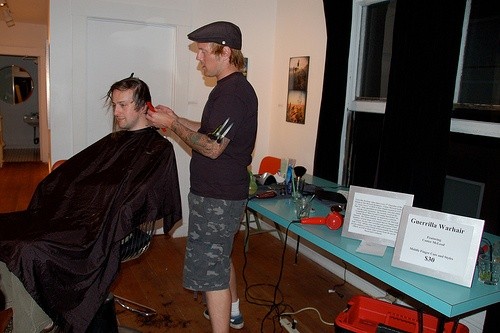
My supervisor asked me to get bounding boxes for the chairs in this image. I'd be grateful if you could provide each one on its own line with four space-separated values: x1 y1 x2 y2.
40 160 156 333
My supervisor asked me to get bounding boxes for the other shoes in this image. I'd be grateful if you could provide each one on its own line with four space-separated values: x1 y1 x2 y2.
203 309 245 329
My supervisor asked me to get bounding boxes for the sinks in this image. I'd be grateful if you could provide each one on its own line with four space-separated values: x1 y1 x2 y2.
23 115 39 127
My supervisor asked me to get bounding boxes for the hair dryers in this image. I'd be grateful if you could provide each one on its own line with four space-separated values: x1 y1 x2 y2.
300 210 344 231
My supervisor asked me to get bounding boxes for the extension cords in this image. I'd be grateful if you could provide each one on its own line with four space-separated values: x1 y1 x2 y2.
280 318 300 333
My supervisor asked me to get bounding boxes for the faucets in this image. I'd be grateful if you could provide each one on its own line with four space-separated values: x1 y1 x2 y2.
32 113 36 116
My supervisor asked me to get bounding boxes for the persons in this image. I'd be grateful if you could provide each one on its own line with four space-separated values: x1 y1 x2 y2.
147 21 258 333
0 73 183 333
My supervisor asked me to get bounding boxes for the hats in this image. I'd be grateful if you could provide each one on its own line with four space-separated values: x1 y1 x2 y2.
187 21 241 50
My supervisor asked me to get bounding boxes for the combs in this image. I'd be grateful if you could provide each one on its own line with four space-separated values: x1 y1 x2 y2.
148 102 166 133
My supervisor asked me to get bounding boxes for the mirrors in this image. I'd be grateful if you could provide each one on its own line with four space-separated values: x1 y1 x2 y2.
0 65 34 104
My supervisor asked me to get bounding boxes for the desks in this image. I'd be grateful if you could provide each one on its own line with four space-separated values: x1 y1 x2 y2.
242 168 500 333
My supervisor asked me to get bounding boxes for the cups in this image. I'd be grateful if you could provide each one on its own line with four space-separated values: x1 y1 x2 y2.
478 254 500 286
291 178 305 198
289 199 312 220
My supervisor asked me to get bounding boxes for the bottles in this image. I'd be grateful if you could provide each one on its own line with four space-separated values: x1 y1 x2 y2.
246 165 258 194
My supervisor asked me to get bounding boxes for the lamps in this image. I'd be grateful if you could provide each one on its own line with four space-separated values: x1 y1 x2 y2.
0 0 16 28
